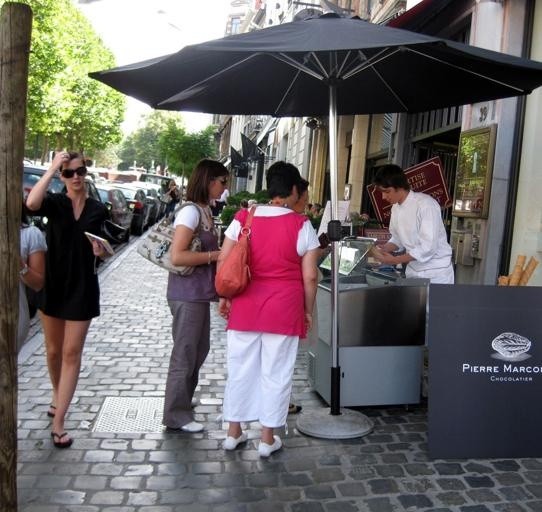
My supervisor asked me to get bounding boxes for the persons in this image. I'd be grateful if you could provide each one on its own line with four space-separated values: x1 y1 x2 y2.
18 194 48 354
26 151 114 448
216 161 321 459
162 160 227 433
157 164 323 415
369 164 456 285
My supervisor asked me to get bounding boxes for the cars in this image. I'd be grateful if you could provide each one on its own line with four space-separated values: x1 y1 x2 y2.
21 167 183 243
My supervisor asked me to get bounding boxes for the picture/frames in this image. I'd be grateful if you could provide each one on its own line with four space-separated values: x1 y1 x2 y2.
452 123 498 219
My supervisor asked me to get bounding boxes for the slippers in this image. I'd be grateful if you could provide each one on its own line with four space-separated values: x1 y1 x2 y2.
51 430 73 448
47 405 57 417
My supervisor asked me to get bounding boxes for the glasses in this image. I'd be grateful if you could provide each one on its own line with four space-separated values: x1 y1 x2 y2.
210 176 228 186
62 166 87 178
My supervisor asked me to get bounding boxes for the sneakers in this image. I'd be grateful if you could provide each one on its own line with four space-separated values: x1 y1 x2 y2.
258 435 283 457
223 431 248 450
288 403 302 415
180 421 204 433
190 396 200 406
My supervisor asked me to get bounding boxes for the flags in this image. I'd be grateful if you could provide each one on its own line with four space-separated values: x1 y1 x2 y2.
231 146 242 167
240 133 260 161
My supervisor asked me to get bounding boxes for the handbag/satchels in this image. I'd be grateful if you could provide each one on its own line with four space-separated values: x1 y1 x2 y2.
137 218 202 277
160 194 173 204
214 234 252 299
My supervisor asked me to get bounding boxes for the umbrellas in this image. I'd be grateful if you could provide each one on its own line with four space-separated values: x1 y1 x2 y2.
89 14 542 414
